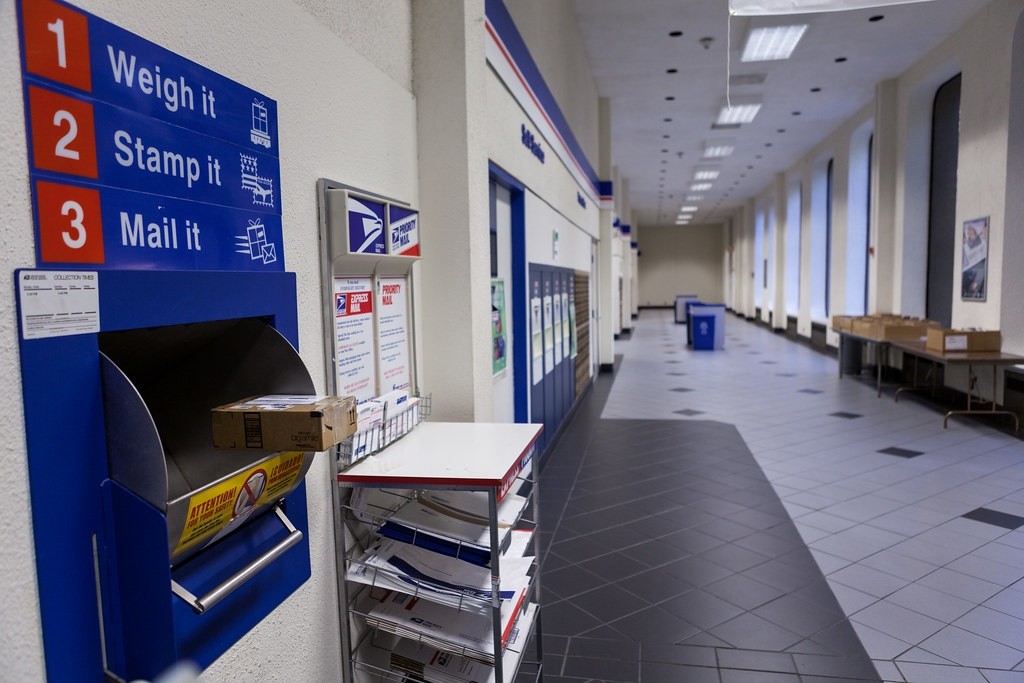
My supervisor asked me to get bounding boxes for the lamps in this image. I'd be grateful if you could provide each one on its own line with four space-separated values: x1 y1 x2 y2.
693 165 721 180
739 15 808 62
715 95 763 123
704 137 734 158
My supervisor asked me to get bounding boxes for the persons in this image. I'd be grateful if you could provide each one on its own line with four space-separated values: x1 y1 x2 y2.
963 222 986 298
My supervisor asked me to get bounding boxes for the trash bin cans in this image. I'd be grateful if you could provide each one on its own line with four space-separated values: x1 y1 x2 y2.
691 315 715 352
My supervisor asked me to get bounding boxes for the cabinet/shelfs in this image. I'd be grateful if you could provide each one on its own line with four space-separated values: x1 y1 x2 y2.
332 421 543 683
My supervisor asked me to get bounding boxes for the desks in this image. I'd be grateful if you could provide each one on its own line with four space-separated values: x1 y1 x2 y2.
673 295 725 351
834 328 1023 430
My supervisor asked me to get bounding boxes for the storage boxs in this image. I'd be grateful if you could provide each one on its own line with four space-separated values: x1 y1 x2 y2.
212 394 358 452
832 314 940 341
926 328 1001 351
